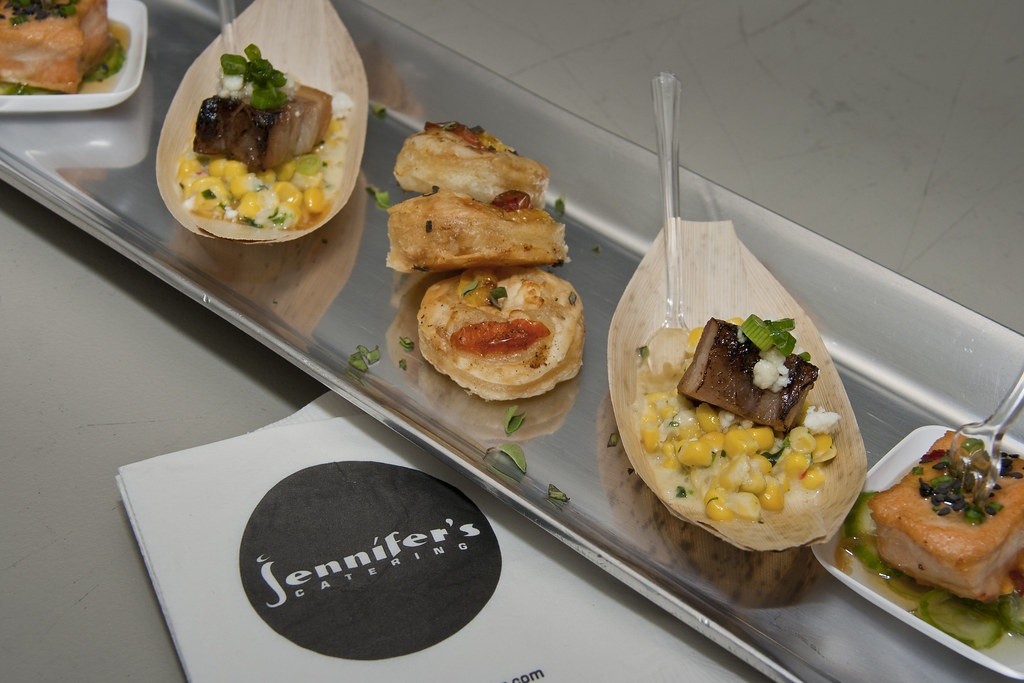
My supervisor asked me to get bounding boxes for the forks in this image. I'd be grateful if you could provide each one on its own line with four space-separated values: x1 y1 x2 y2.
945 369 1024 504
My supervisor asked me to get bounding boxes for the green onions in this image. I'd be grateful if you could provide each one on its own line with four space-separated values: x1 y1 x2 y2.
740 313 810 362
223 43 289 111
0 0 75 24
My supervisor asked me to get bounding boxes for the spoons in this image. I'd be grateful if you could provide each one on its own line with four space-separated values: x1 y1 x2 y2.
636 70 694 401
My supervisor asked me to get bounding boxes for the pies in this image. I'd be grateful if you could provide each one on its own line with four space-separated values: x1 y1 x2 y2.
385 120 585 401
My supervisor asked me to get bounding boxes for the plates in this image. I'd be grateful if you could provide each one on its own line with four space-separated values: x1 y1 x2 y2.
0 72 152 168
167 171 367 344
606 218 868 553
597 402 820 607
809 422 1023 680
155 1 370 242
0 1 148 114
